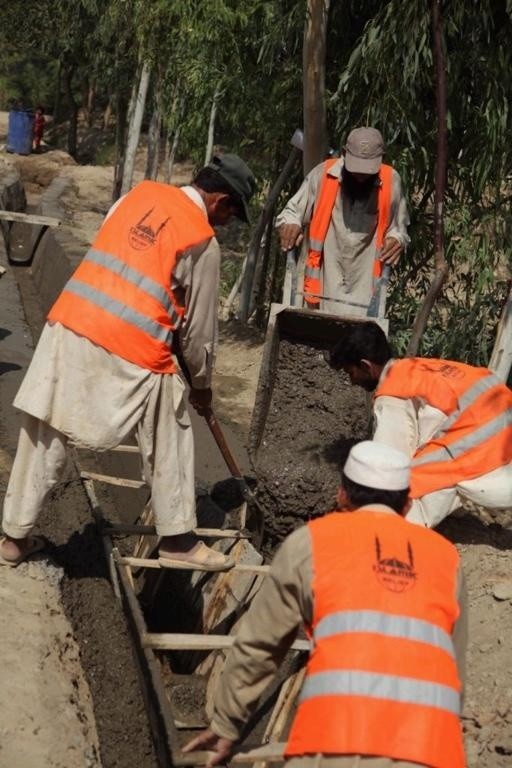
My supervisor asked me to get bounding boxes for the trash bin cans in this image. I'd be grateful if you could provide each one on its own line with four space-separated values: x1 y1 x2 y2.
7 109 36 154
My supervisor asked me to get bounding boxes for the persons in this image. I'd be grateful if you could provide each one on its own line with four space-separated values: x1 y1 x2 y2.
325 320 512 530
0 153 259 571
182 440 469 768
33 106 46 149
274 127 411 317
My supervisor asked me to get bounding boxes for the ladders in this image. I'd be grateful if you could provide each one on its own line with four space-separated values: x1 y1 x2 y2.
70 435 319 768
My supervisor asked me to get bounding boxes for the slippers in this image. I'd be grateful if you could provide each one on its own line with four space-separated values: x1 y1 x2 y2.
156 538 236 573
0 534 46 568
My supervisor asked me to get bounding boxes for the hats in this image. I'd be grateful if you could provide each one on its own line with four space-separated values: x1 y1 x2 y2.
342 439 413 493
207 151 257 230
342 126 385 175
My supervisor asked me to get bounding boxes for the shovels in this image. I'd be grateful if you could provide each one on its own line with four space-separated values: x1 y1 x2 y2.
170 330 267 553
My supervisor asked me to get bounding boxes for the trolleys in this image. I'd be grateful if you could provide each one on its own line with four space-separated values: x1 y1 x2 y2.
246 234 459 528
